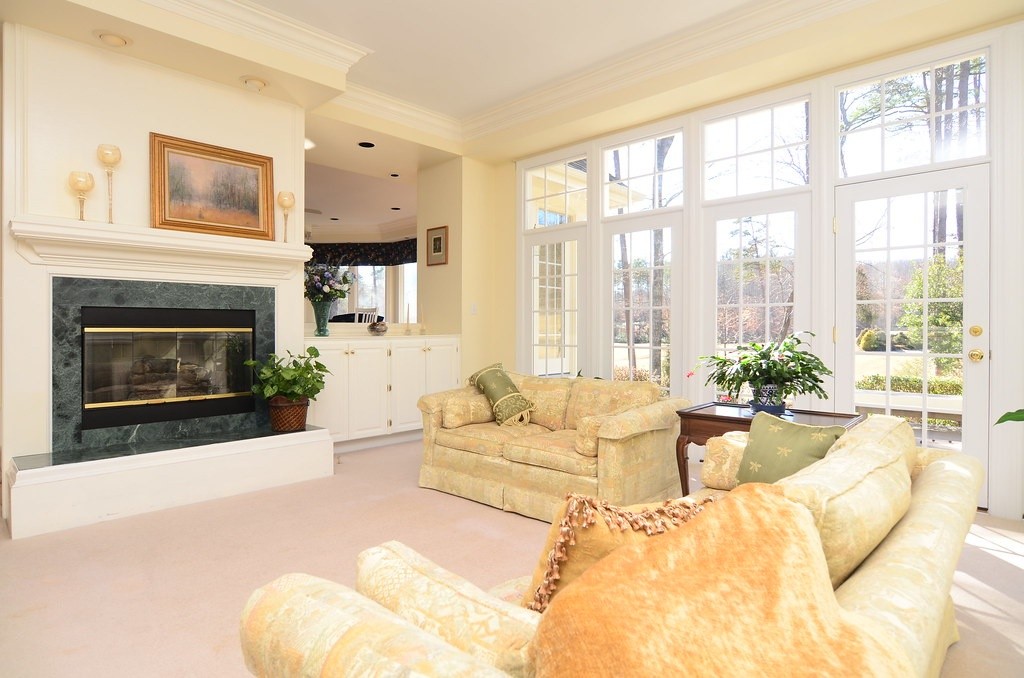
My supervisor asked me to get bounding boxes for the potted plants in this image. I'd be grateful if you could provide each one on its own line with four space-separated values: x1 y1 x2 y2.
242 346 334 432
686 330 834 417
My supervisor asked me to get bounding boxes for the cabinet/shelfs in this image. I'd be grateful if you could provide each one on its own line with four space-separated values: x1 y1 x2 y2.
303 334 461 443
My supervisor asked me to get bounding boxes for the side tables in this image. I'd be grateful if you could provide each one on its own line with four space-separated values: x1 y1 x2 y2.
675 401 795 498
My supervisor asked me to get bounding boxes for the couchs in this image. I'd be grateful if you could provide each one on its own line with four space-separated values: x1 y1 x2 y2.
418 373 693 525
240 414 978 678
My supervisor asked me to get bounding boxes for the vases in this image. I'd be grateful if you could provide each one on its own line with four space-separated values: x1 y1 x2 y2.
312 301 330 337
366 322 388 336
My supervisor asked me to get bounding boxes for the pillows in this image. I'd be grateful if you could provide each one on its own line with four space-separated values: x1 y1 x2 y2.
527 482 865 678
468 361 537 427
738 410 847 485
574 404 646 457
519 490 722 617
441 392 495 429
700 436 746 491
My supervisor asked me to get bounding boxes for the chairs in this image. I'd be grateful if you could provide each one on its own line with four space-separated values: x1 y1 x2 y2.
354 306 379 324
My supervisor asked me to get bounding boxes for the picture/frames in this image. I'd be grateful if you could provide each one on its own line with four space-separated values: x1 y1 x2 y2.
426 224 450 267
148 130 275 241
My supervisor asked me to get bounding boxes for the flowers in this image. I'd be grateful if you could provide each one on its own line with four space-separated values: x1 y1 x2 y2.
304 253 364 335
